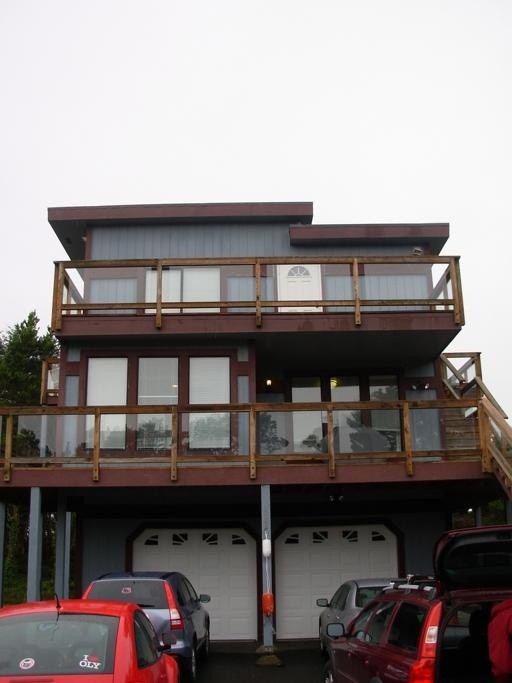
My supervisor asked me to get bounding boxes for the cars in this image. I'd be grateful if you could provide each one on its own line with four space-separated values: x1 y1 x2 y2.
316 579 398 659
83 571 210 681
2 594 179 683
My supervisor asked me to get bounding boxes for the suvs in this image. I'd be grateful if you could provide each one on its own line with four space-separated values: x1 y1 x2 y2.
321 525 511 683
